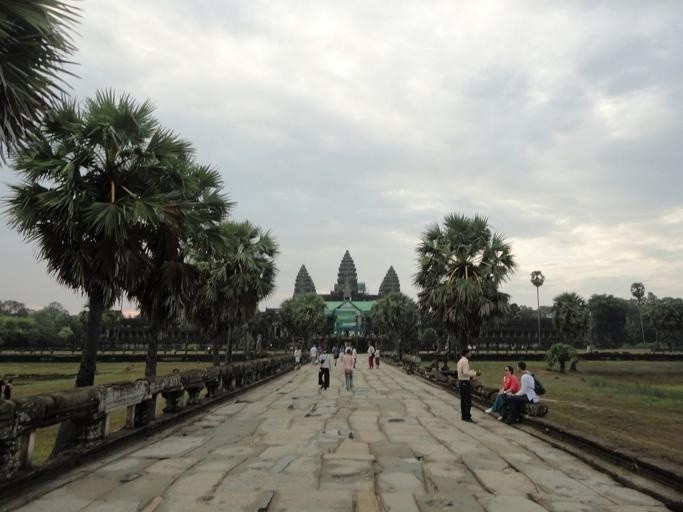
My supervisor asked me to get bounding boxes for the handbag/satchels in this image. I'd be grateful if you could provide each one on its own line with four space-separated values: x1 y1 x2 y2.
320 360 324 364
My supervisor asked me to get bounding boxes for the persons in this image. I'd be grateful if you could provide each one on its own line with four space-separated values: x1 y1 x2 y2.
366 340 374 368
374 346 379 368
341 348 354 391
457 348 481 423
317 348 330 391
484 365 520 421
502 361 535 425
293 340 358 370
0 375 12 399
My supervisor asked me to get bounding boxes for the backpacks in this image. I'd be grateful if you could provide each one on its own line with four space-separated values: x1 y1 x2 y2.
521 372 545 395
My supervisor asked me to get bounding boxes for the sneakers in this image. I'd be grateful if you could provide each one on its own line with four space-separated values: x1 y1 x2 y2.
319 385 354 391
461 406 522 425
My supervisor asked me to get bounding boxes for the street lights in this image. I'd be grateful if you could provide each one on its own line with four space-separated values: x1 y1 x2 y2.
630 281 647 345
529 269 546 347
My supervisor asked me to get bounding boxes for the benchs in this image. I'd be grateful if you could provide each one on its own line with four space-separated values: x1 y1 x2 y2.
470 385 548 418
